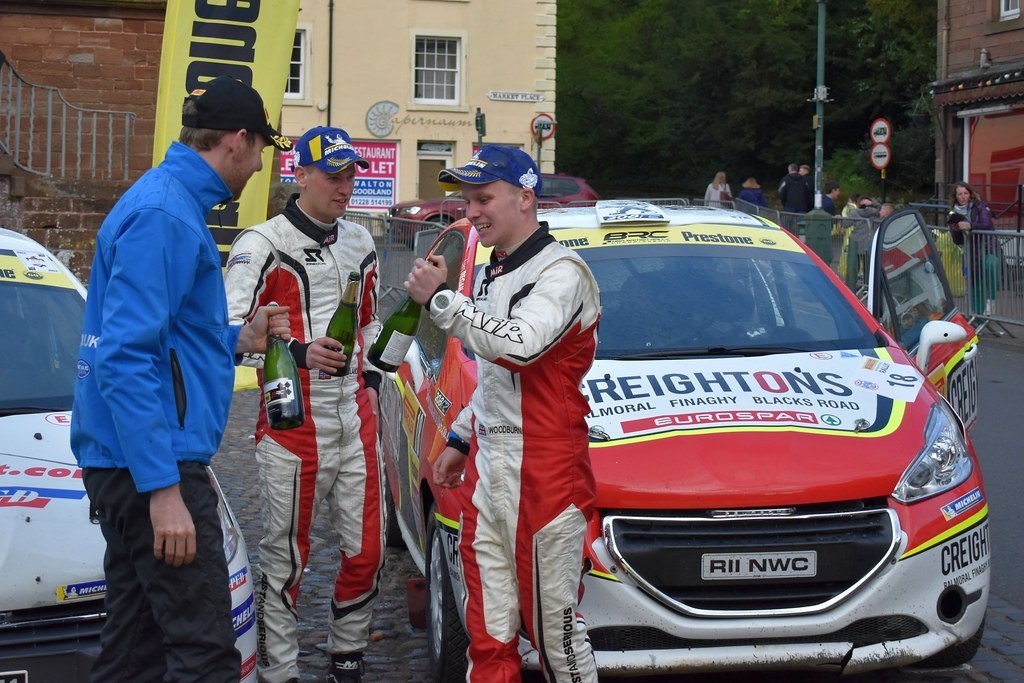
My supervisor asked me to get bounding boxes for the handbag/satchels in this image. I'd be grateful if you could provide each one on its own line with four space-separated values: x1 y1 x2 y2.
720 192 734 207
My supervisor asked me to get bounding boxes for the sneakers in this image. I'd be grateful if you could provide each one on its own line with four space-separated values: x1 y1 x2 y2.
330 653 365 683
984 299 997 315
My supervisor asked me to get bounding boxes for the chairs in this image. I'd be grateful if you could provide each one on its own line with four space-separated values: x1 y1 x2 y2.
638 266 758 349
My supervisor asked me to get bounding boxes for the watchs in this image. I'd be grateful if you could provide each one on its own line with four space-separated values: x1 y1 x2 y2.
429 289 457 317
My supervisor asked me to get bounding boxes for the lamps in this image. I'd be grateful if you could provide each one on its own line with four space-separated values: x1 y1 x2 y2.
977 47 991 69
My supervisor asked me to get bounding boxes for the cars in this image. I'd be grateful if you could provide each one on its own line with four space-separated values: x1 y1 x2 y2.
0 226 258 683
376 198 990 676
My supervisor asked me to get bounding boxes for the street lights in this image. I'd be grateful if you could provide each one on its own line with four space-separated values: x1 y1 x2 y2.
535 119 557 173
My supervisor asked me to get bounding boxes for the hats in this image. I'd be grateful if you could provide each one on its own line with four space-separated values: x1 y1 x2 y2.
291 126 370 174
439 144 542 199
181 76 293 152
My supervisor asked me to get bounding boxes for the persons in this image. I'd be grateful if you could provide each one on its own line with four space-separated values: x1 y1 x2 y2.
877 202 895 218
839 195 880 290
945 181 1004 334
222 124 388 682
797 163 817 204
820 181 839 217
402 143 602 683
777 163 811 235
703 172 735 210
738 177 768 208
67 74 299 682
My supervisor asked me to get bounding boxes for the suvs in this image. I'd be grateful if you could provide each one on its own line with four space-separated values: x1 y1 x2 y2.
389 174 602 251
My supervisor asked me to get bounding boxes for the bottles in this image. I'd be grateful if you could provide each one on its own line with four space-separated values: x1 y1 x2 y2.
368 260 442 372
326 271 362 376
263 301 306 430
